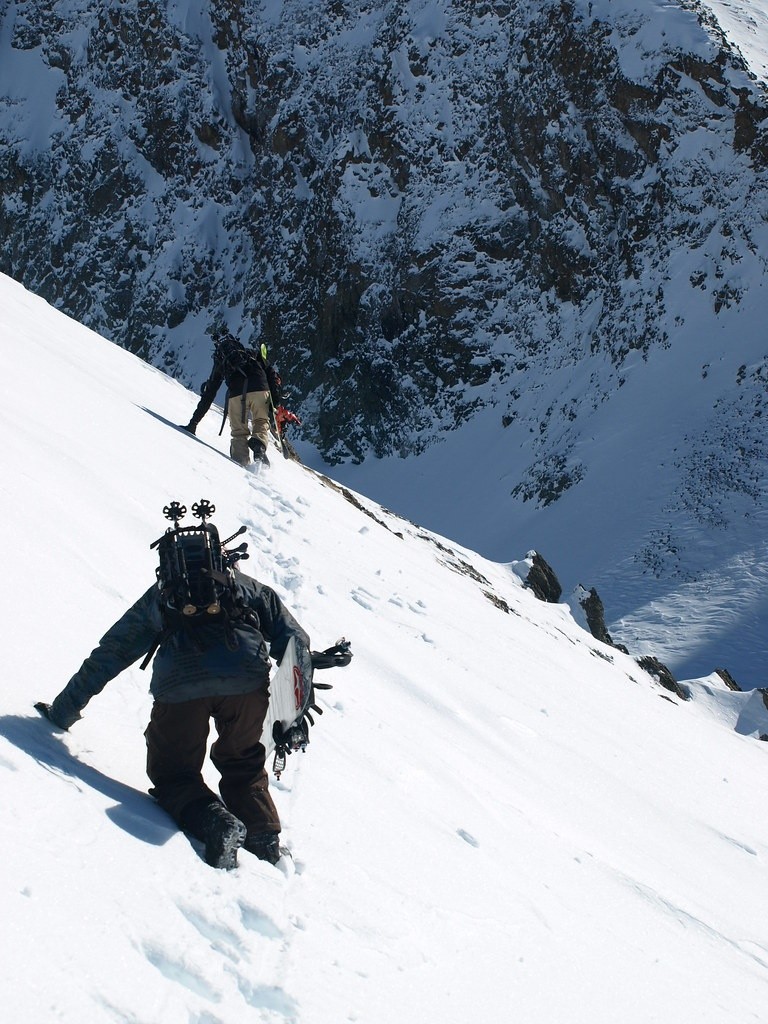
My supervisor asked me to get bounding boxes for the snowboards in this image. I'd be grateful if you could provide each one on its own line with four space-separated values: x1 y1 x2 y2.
260 344 301 460
259 636 354 781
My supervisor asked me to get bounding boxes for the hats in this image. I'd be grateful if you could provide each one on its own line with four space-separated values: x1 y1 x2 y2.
525 550 537 559
222 548 231 568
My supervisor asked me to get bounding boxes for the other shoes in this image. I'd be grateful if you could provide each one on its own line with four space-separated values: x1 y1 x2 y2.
247 833 280 862
236 456 250 467
197 801 246 871
253 446 270 467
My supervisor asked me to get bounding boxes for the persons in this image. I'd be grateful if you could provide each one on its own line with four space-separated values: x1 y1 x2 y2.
34 522 310 871
181 337 280 467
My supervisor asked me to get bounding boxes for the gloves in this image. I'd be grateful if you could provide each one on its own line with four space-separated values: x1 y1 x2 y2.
272 721 298 746
33 702 82 729
180 418 197 434
